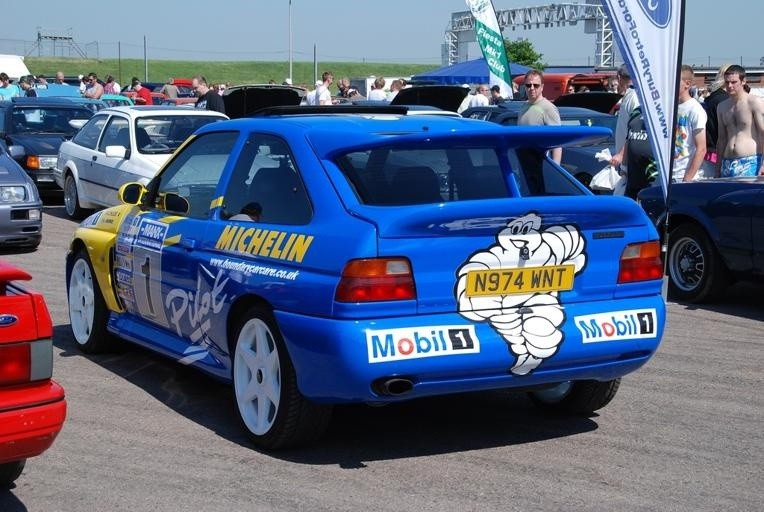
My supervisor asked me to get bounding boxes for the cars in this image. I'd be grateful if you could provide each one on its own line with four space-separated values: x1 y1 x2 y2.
53 105 232 221
56 97 670 446
637 176 763 306
121 71 626 195
1 96 96 197
1 248 70 488
0 145 44 253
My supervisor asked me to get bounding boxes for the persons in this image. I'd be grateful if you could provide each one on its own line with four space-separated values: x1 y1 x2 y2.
607 67 643 197
570 66 763 133
1 72 153 106
701 65 751 178
516 67 565 171
224 201 265 224
270 71 407 106
670 63 708 184
457 84 504 114
623 106 656 199
714 64 764 178
160 73 229 114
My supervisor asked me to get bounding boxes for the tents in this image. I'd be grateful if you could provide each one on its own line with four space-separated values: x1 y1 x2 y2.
411 57 530 87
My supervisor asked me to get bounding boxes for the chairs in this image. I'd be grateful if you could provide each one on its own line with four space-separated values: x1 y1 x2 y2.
370 166 442 205
247 166 305 222
448 164 521 199
115 128 149 149
14 112 61 132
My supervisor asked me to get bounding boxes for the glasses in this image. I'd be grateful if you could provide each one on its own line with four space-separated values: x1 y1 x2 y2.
525 84 540 88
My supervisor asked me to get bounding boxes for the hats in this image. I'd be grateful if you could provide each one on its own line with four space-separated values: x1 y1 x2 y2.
18 76 29 84
169 78 174 84
490 85 500 91
57 72 64 79
711 64 732 92
347 89 355 95
285 79 293 85
